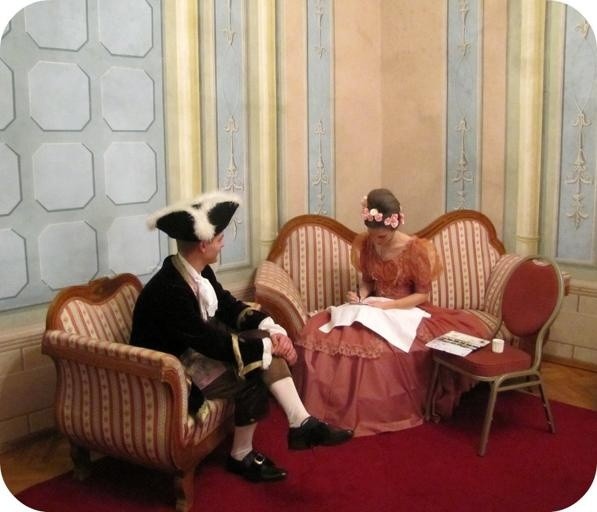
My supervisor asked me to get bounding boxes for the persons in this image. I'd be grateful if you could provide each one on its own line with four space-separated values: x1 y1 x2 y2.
295 188 492 439
129 190 355 480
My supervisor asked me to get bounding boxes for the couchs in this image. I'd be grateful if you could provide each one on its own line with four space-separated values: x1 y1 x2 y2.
254 210 571 400
40 273 262 512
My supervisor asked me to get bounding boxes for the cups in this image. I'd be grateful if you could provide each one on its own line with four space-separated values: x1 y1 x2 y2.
491 339 504 353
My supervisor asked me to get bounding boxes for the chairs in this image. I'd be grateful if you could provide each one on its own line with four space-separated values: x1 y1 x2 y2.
425 255 564 456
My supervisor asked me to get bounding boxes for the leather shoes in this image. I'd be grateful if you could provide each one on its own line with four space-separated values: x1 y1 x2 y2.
287 415 353 449
226 449 287 481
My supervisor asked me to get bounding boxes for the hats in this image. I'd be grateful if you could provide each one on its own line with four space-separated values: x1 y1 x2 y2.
147 191 239 241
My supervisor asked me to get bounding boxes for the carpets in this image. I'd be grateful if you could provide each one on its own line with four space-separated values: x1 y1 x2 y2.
15 383 596 512
